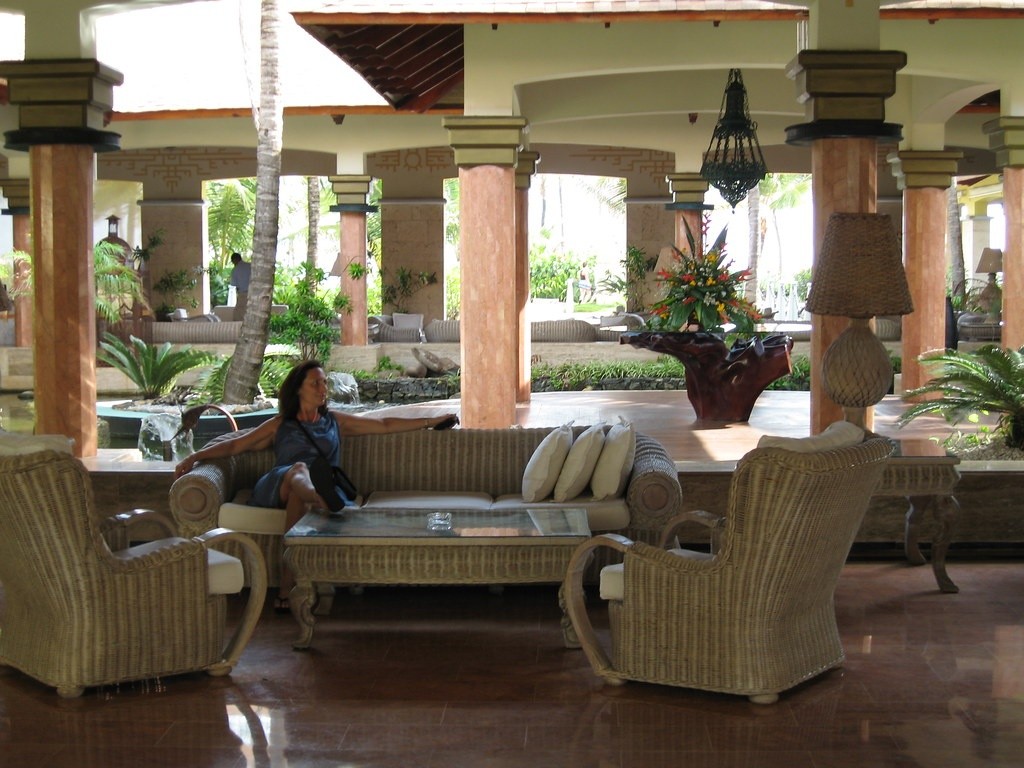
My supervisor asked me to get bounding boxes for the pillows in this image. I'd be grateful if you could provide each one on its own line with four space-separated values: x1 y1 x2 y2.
603 420 637 501
521 420 575 504
554 420 606 503
586 415 630 502
391 314 423 329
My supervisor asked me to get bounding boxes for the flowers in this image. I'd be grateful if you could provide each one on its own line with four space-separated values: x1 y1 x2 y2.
645 212 764 334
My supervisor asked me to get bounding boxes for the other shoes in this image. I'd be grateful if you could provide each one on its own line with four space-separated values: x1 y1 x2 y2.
272 596 291 614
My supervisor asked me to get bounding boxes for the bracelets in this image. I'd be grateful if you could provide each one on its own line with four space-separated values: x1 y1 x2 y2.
425 418 429 428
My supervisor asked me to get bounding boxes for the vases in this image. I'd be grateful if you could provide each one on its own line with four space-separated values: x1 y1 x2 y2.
683 310 708 331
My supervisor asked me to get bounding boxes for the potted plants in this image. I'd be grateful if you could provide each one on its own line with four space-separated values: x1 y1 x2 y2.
152 300 177 322
378 263 440 328
152 263 219 323
592 245 659 331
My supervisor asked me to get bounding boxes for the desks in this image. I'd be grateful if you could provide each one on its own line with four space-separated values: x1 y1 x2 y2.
871 439 962 595
959 324 1003 342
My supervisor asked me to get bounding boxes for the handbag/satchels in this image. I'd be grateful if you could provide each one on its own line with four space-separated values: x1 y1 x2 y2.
311 466 358 512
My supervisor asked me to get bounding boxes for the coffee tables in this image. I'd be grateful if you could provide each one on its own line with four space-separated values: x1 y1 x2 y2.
284 507 592 649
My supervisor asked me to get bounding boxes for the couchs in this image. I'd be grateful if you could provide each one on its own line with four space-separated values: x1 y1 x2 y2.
422 318 598 344
565 419 899 705
600 307 655 329
0 427 268 700
169 423 683 608
369 313 425 343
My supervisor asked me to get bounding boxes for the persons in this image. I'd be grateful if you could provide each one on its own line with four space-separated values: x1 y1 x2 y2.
231 253 251 321
174 360 460 613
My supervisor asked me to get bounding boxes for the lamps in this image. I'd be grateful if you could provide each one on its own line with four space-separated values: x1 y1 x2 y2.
975 248 1003 325
698 69 774 215
803 211 915 442
